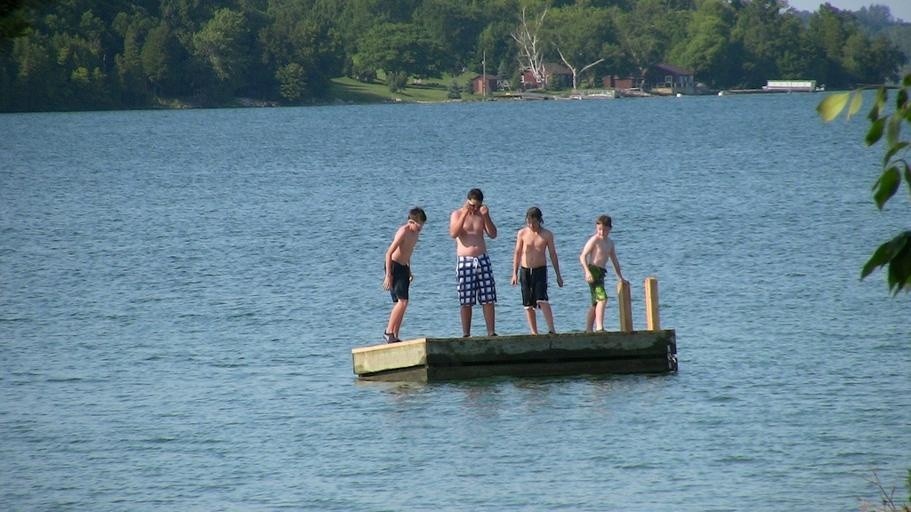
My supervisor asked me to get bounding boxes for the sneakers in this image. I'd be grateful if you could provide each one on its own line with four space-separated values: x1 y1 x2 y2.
383 329 400 343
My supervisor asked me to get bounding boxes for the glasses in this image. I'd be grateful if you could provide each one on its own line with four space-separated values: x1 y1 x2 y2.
467 200 482 207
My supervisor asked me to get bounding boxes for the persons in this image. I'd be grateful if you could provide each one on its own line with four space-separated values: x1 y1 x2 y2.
578 214 630 333
448 187 499 338
379 205 427 346
509 206 564 335
